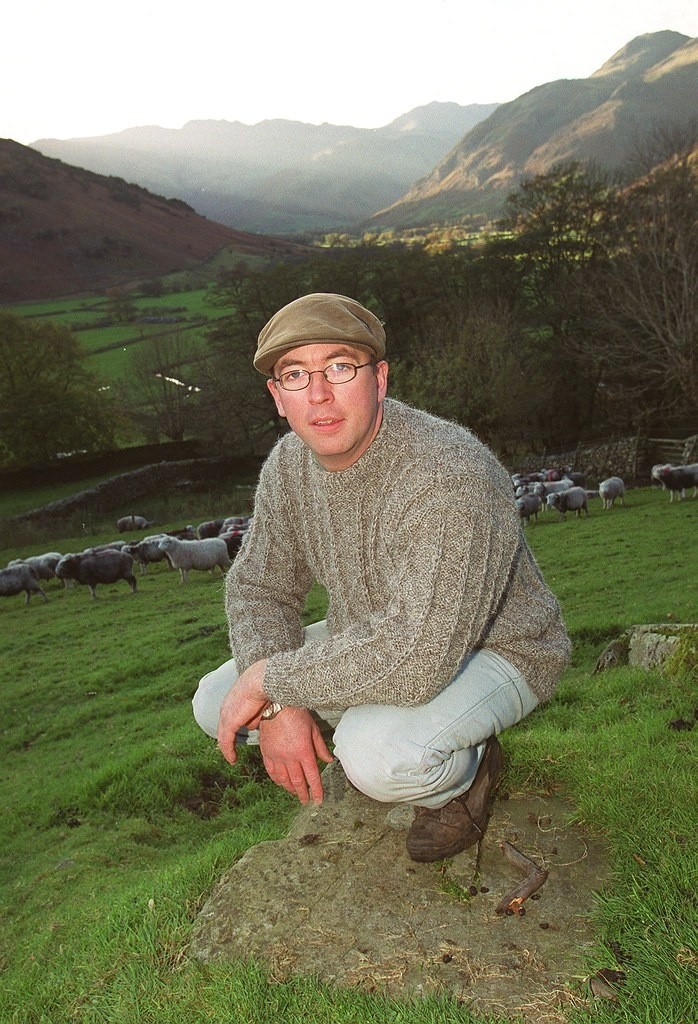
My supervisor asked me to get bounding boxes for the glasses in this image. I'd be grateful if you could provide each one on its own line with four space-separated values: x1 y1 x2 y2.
273 362 370 391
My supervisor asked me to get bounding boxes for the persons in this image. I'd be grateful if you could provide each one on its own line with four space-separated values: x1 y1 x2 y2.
190 291 576 864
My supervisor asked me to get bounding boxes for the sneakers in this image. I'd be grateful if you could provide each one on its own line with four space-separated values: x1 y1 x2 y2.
405 734 505 860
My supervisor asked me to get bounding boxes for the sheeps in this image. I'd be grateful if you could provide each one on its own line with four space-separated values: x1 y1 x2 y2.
117 516 154 535
545 486 588 521
83 535 175 576
598 477 625 510
55 550 137 600
167 516 255 558
0 564 50 605
515 493 543 528
512 467 585 512
7 551 71 591
651 463 698 503
158 533 232 585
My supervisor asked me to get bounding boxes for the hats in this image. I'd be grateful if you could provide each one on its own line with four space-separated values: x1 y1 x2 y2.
252 293 386 376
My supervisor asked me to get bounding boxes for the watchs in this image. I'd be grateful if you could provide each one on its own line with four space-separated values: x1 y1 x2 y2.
259 701 285 722
